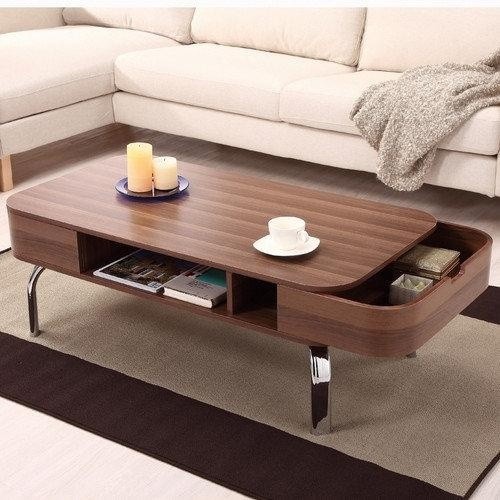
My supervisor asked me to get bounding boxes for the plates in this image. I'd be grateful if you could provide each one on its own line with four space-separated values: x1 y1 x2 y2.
252 233 322 257
114 174 191 199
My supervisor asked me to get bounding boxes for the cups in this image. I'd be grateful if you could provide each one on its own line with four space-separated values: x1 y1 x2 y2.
267 215 310 252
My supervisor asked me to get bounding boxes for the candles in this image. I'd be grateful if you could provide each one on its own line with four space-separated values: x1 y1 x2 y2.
126 141 178 193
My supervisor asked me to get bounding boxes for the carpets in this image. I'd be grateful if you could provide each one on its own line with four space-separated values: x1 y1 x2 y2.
0 247 500 500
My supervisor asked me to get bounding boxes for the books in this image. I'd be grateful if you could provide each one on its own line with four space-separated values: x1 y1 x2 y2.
92 244 194 295
163 265 227 309
